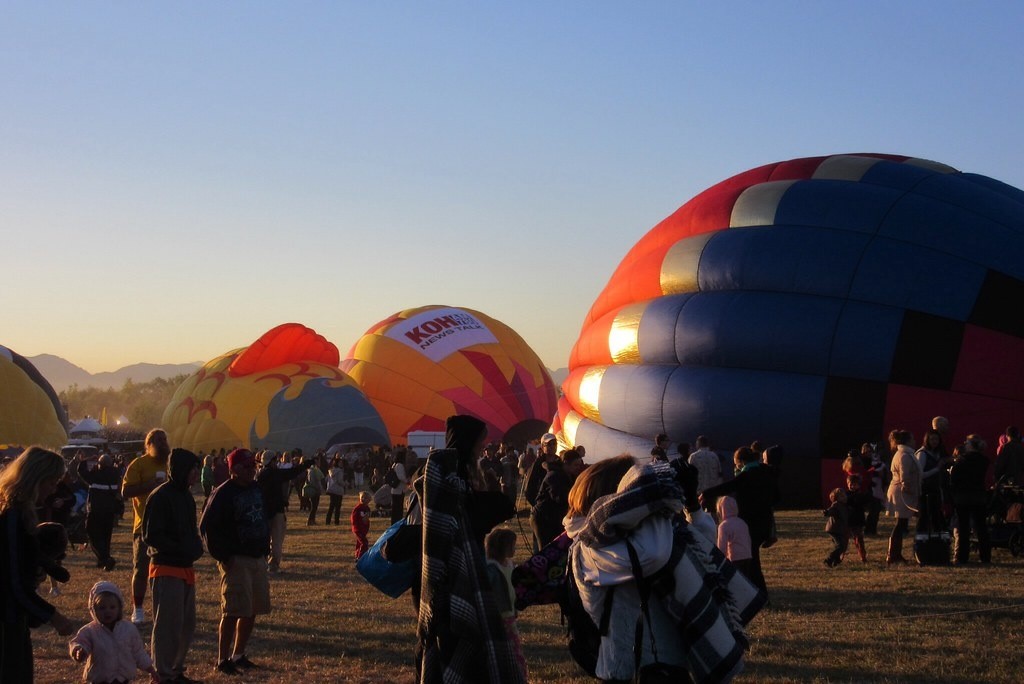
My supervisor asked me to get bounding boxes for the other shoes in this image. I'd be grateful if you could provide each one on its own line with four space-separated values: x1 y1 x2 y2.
97 558 116 571
306 517 318 527
823 556 843 568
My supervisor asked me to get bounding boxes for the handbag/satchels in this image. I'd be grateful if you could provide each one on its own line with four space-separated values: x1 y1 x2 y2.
914 537 953 566
385 469 401 489
301 482 317 497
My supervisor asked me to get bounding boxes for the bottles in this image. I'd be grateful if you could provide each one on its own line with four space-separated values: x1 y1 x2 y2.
268 556 283 578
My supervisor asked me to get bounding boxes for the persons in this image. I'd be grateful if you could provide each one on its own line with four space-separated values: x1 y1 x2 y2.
821 416 1024 569
0 416 789 684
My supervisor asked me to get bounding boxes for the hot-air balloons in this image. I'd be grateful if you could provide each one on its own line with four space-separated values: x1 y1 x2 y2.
159 323 390 470
337 305 560 456
544 151 1024 520
0 343 72 452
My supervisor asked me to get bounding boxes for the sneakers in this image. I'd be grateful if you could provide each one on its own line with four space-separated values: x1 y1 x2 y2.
131 607 144 624
214 655 260 676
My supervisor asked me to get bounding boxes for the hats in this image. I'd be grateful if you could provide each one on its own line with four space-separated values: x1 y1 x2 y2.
260 450 276 465
541 433 557 444
228 448 262 469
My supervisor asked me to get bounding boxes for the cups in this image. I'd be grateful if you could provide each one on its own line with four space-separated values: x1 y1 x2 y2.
155 471 166 482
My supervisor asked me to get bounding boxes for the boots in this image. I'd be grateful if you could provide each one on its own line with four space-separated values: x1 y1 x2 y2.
326 510 341 525
886 536 909 564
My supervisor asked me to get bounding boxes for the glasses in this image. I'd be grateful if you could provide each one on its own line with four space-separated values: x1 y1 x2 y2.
662 439 670 442
